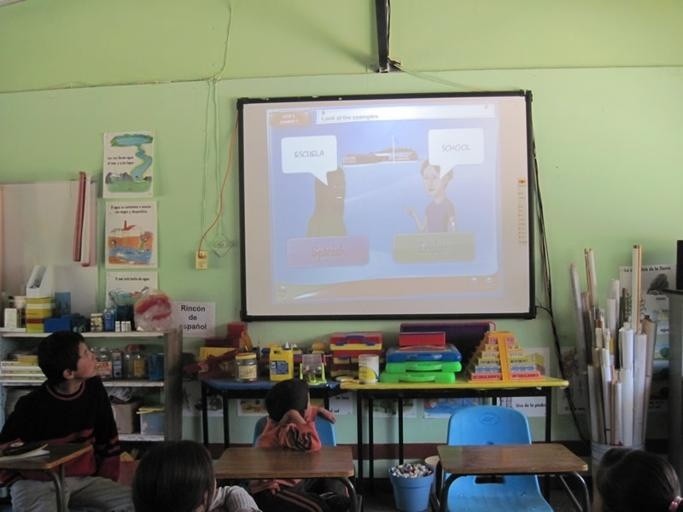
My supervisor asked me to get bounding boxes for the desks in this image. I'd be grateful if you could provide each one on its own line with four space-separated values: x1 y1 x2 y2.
338 377 569 496
0 443 93 512
195 373 339 488
434 444 592 512
213 446 358 512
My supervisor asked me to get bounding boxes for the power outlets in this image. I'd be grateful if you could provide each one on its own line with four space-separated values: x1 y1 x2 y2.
195 250 207 270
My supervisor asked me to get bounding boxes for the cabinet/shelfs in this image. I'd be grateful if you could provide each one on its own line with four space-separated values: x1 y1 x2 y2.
0 326 181 461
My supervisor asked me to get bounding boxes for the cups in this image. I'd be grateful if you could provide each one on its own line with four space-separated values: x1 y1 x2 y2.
114 320 131 332
357 354 379 384
301 353 322 378
107 228 140 253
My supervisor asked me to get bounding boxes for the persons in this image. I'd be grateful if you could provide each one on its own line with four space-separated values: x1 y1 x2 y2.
128 435 261 509
0 329 135 509
246 377 341 511
592 443 681 510
402 158 455 233
299 167 347 236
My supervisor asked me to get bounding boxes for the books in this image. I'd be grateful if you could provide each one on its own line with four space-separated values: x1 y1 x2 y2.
0 440 50 466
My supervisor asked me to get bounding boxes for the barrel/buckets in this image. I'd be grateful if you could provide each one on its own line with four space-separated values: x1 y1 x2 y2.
269 345 295 381
389 462 435 510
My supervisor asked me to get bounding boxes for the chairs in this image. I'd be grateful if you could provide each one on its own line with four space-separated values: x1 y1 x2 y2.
443 405 553 512
254 415 334 447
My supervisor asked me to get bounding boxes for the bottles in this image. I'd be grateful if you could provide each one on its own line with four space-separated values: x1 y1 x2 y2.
90 344 146 379
203 321 247 348
89 312 103 333
103 305 116 331
234 353 258 383
